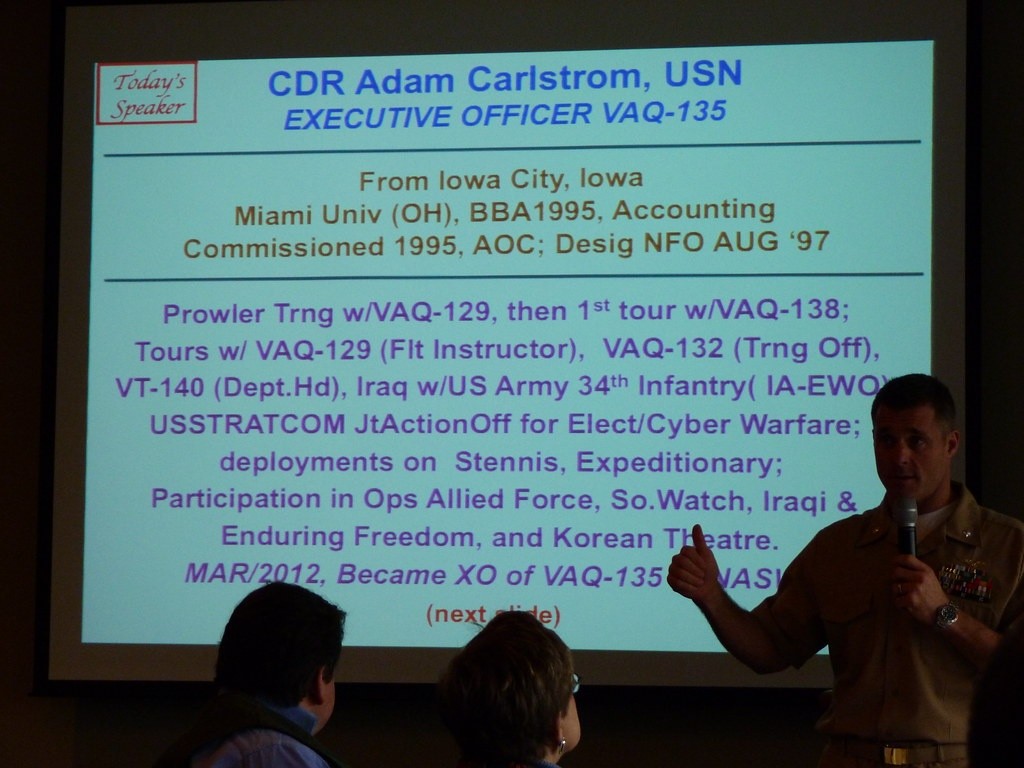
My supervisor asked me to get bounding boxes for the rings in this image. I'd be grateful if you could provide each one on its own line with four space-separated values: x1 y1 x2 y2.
896 583 903 596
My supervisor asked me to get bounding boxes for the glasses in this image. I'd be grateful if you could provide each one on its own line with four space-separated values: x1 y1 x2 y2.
568 673 583 693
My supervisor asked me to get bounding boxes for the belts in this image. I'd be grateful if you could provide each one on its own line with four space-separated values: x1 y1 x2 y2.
828 735 968 765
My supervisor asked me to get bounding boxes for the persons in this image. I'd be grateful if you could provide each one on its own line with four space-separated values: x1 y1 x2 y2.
153 582 355 768
667 373 1024 768
439 611 582 768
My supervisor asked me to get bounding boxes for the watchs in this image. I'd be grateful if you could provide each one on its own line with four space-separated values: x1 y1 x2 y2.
934 601 959 633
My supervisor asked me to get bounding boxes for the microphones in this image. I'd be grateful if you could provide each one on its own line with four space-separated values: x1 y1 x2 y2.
894 495 916 562
